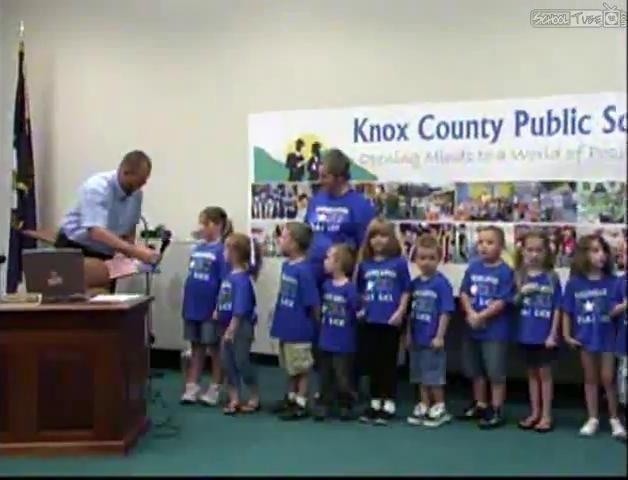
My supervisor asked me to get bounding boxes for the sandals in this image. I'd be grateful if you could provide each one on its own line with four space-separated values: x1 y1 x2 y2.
519 416 552 432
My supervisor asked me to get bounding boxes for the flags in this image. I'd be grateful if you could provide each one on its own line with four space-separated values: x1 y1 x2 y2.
5 51 38 295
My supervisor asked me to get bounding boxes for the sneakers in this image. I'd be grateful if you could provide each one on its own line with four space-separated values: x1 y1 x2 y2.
407 400 504 430
580 418 600 436
182 382 259 416
272 392 396 425
610 417 627 438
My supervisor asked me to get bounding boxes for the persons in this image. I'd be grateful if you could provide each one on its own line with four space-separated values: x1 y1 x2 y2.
357 218 414 427
512 228 564 435
609 234 628 409
402 234 457 429
267 220 322 423
211 231 265 417
314 241 365 423
284 139 307 181
250 181 309 220
456 223 518 431
303 147 375 404
180 204 235 409
380 186 578 261
306 142 324 182
560 234 628 443
51 148 164 296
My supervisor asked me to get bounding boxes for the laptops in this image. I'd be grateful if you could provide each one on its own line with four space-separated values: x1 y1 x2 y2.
21 248 96 303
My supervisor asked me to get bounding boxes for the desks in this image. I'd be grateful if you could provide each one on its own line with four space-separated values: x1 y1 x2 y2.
0 295 155 456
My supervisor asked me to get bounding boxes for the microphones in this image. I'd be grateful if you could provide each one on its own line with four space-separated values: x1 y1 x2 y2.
160 238 170 253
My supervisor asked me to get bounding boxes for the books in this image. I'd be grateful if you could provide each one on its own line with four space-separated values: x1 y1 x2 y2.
102 256 160 283
